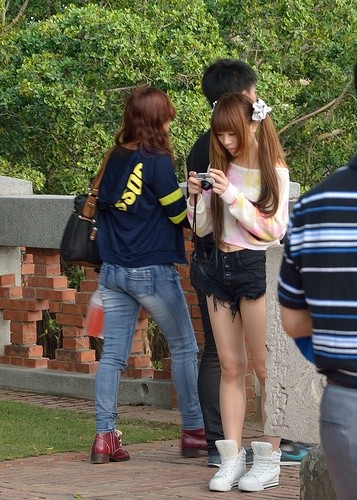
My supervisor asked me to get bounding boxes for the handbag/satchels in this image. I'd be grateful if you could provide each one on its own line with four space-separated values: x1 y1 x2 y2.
83 288 107 339
60 146 117 268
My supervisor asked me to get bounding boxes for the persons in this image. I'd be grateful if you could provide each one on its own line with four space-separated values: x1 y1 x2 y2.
186 57 311 466
277 63 357 500
187 93 289 491
90 86 208 465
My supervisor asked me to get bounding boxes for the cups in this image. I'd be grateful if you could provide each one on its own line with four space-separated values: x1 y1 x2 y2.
86 298 104 337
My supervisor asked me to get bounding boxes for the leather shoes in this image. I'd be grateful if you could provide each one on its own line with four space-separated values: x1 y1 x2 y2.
92 429 129 463
181 428 208 456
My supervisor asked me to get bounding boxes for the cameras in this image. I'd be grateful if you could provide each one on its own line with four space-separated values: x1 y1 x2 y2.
195 173 216 190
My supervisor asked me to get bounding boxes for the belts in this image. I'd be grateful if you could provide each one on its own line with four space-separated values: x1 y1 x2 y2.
326 378 342 386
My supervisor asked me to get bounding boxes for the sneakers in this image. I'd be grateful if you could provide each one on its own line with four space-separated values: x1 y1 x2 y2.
209 440 247 492
208 447 253 466
238 440 282 492
278 445 310 465
